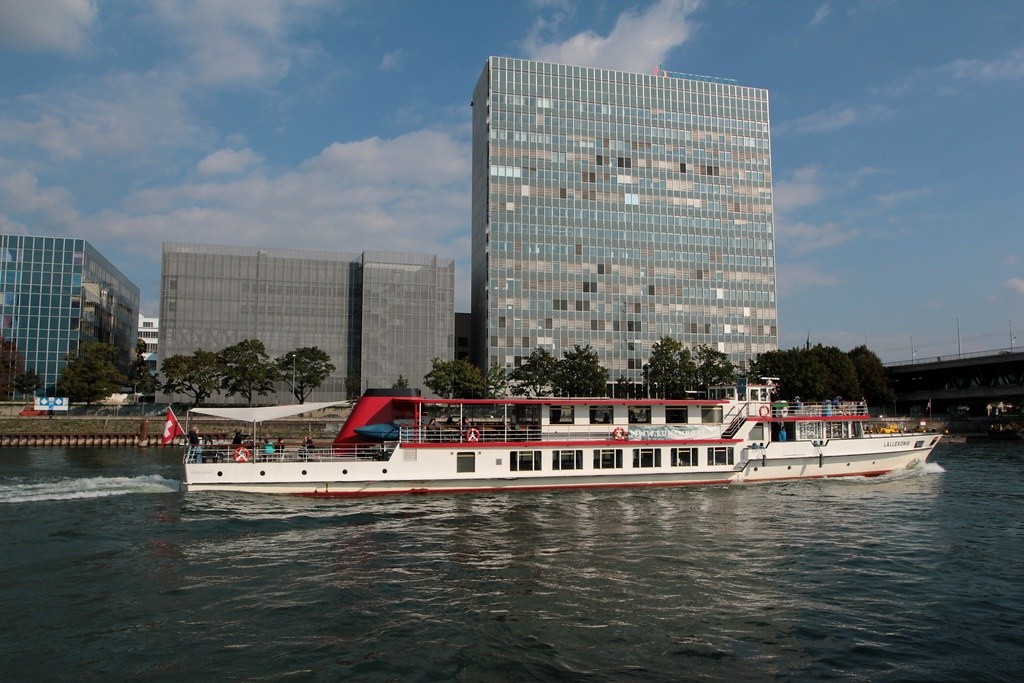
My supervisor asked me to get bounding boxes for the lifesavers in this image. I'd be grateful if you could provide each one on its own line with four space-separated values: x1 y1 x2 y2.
234 447 250 463
759 405 770 417
466 428 480 443
613 427 626 440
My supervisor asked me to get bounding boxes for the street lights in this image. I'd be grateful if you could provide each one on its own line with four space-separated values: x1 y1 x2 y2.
1008 319 1016 351
957 314 962 359
910 335 917 364
292 354 296 404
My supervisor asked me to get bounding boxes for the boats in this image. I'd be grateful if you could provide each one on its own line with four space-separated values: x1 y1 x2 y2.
178 375 948 497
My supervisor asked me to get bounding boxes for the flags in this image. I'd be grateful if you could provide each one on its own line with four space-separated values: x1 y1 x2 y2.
160 407 184 448
926 397 931 412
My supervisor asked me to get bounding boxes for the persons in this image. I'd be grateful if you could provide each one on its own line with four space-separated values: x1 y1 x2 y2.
261 436 316 462
185 427 200 460
770 387 802 442
232 427 242 445
603 412 610 423
418 415 472 439
